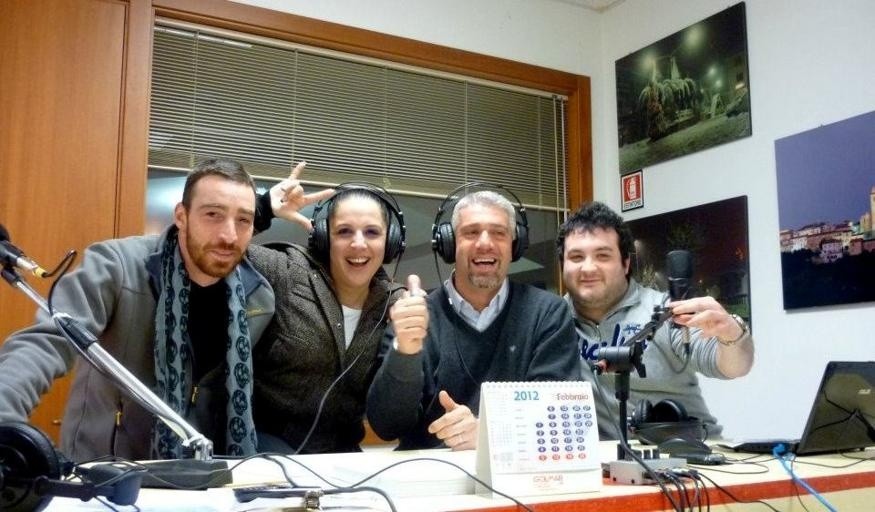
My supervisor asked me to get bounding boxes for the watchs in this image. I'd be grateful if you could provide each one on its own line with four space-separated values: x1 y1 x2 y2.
719 312 751 348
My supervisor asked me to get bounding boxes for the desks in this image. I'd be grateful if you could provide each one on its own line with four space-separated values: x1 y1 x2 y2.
47 440 875 512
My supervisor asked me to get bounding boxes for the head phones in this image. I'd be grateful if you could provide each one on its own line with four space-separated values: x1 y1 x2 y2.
625 398 709 450
307 179 408 267
1 423 144 511
429 181 531 263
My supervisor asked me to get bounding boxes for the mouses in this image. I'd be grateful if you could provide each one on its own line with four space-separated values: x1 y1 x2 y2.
663 433 713 465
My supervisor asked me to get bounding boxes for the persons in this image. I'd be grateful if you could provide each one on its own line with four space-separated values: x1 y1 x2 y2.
554 200 756 441
647 86 667 142
0 159 278 466
243 160 408 454
365 179 583 453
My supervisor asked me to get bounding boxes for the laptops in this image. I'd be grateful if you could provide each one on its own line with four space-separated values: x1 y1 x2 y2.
734 360 874 456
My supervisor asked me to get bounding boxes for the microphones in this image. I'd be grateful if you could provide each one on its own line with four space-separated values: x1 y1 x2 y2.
667 248 695 353
0 223 51 286
